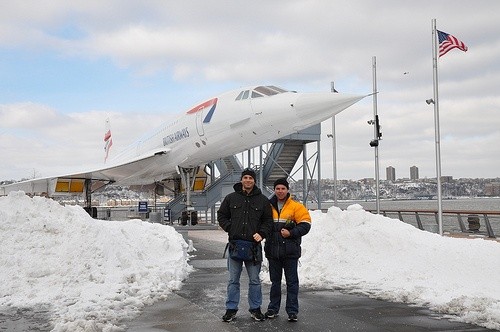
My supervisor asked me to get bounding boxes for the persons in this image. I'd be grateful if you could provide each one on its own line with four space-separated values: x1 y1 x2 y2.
217 167 274 322
264 178 311 321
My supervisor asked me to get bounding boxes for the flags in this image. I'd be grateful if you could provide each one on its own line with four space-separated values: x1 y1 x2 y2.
104 130 112 151
433 29 468 58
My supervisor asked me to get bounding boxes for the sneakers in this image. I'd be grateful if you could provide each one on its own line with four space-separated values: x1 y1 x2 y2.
288 313 298 321
264 310 279 318
249 308 266 321
222 309 238 322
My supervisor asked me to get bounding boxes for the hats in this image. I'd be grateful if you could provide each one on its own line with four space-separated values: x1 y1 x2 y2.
241 167 257 183
274 178 289 190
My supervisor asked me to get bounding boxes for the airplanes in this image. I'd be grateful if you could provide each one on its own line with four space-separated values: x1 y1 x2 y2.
1 83 379 226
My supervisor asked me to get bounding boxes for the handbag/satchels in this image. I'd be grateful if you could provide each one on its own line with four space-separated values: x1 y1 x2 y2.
223 240 258 262
